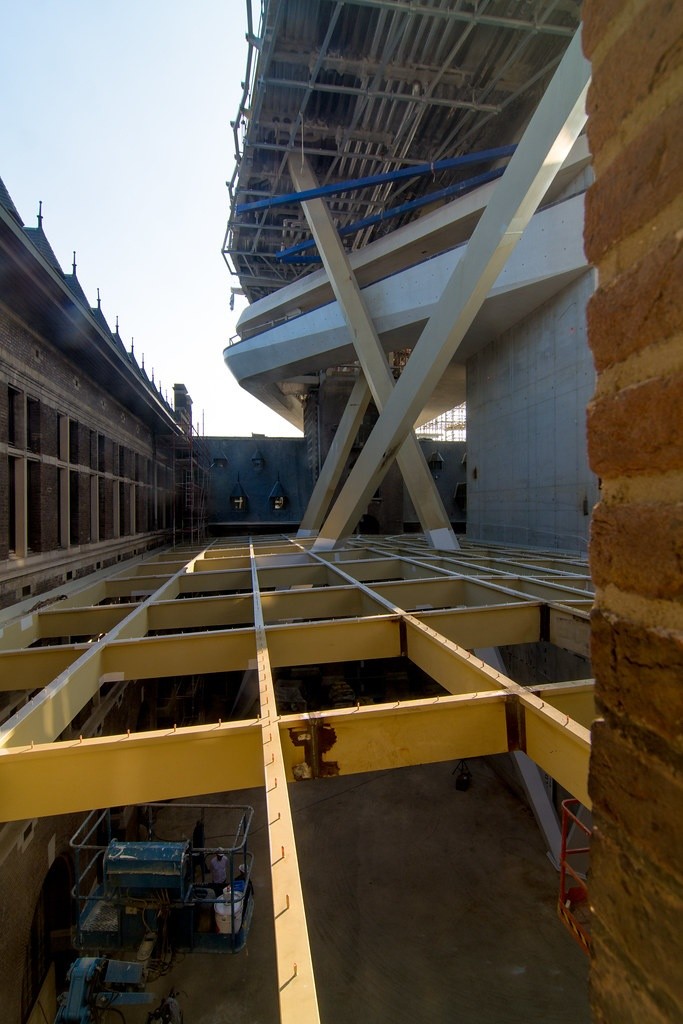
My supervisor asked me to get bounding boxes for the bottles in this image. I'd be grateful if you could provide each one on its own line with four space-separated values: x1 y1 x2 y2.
223 887 232 906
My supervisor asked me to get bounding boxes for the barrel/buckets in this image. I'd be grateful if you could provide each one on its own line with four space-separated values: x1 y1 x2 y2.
214 891 243 933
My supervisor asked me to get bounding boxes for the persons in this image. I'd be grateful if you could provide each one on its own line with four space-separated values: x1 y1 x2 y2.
208 847 228 897
235 864 254 917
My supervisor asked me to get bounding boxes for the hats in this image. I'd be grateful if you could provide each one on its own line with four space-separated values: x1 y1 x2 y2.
216 846 225 856
238 864 248 873
